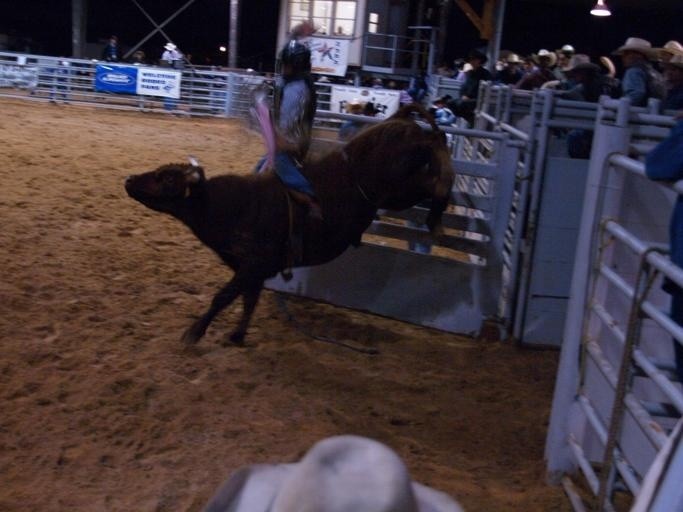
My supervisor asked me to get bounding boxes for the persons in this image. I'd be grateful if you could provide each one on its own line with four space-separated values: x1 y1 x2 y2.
159 43 182 111
248 40 326 233
102 35 119 63
128 51 149 64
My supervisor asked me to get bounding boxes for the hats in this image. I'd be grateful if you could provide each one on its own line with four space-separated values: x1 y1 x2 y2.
199 434 465 512
617 37 651 56
532 49 557 66
164 42 177 51
501 53 526 63
457 63 473 73
647 40 683 63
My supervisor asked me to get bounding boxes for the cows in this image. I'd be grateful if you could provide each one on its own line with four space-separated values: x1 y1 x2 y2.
124 103 457 347
